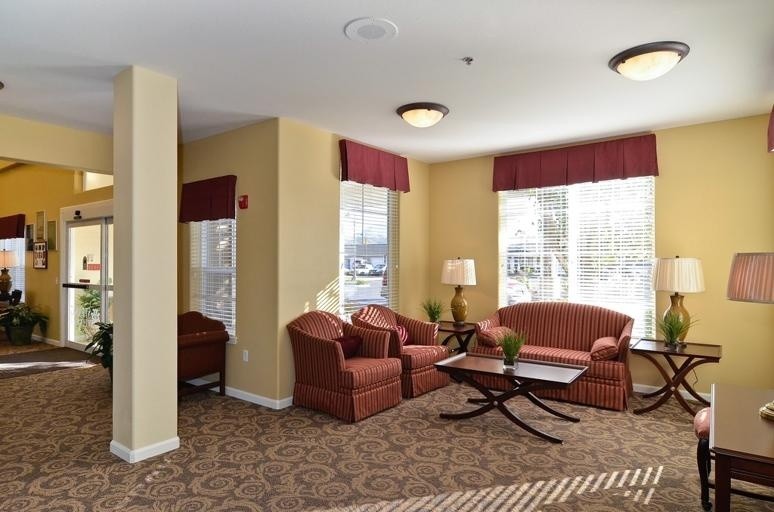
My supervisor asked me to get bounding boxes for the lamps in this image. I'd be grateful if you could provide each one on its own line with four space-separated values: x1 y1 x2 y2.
727 253 774 421
652 256 706 348
608 42 691 83
396 102 450 129
332 334 362 359
1 248 19 299
441 256 476 328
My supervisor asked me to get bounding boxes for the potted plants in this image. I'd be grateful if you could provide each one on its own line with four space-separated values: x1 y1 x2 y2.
650 312 699 351
83 322 114 383
421 300 454 327
0 302 49 345
498 330 525 371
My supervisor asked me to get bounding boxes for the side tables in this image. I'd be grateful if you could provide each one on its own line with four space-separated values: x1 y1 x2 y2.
431 321 476 355
629 338 723 418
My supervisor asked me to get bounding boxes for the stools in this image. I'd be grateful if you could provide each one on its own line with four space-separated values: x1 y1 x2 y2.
694 407 774 512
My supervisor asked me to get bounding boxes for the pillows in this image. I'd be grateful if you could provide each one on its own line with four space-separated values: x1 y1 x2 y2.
392 326 412 345
591 336 618 361
479 327 515 347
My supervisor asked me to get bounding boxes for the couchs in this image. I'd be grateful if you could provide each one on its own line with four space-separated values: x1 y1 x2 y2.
286 311 402 422
178 311 228 416
469 303 634 411
352 304 449 398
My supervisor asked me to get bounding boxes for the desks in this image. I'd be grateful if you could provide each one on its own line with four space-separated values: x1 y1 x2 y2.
712 382 774 512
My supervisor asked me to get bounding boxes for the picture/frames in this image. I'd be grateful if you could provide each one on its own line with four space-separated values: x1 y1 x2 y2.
25 224 34 252
35 211 45 242
32 241 47 269
47 220 56 252
